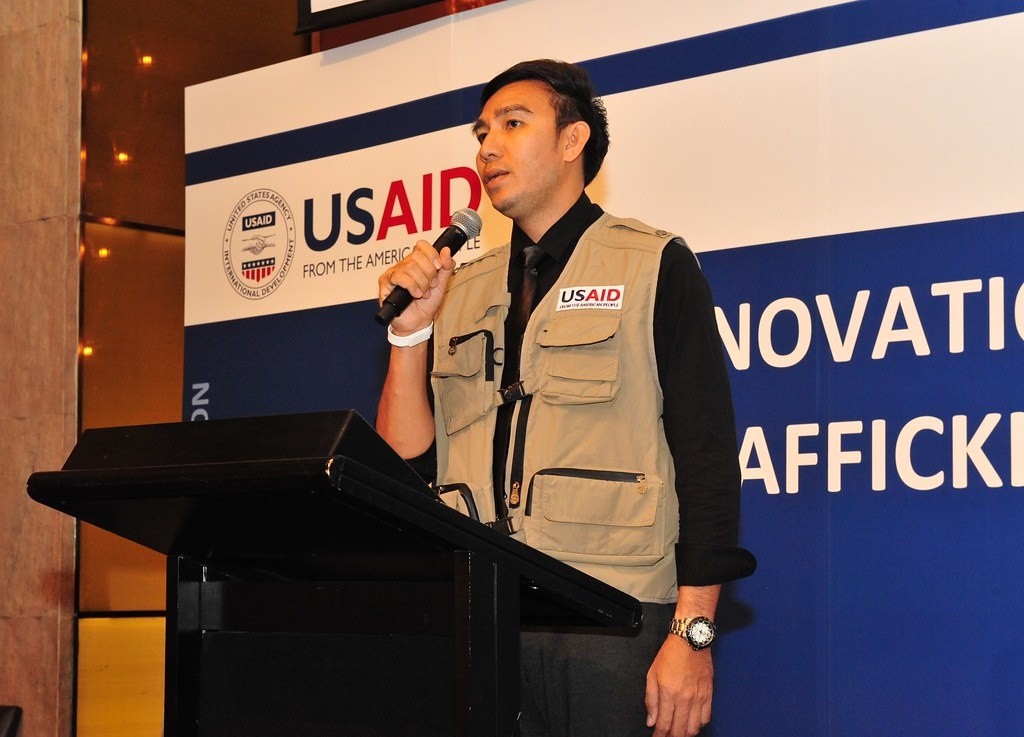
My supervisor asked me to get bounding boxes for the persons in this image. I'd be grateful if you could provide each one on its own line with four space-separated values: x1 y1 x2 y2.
374 59 742 737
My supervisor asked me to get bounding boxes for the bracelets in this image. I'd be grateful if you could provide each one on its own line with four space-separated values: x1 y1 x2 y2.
388 321 434 347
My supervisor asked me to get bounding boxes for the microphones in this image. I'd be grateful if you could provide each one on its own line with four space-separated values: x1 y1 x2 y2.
375 208 482 326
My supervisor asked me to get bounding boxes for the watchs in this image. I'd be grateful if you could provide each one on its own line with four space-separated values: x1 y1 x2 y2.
667 615 719 652
